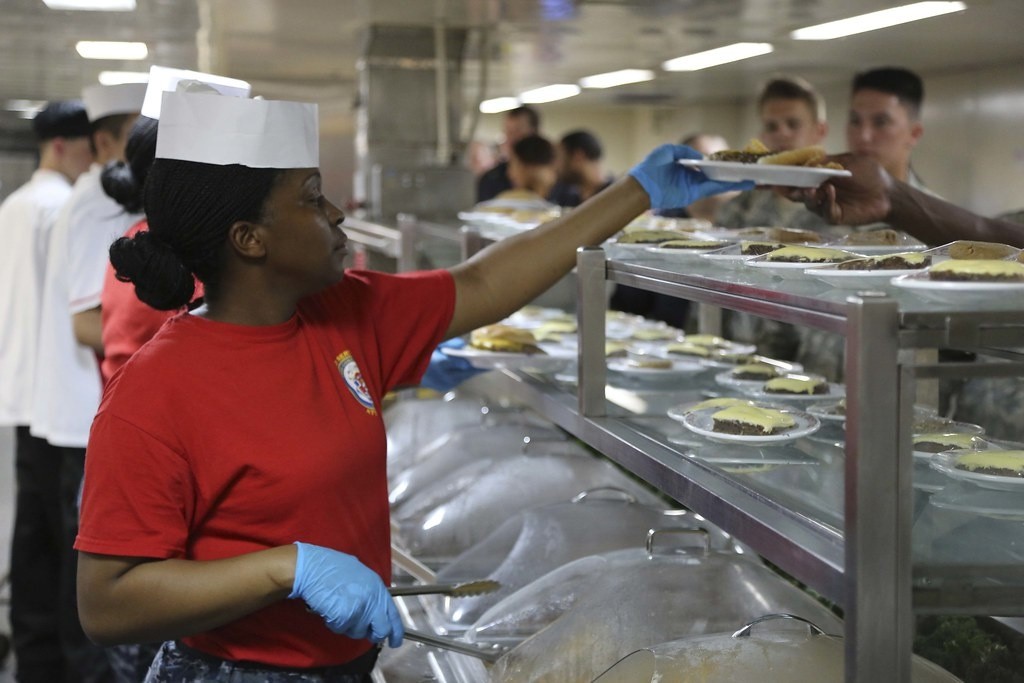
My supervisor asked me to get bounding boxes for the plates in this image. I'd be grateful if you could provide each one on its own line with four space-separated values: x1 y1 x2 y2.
438 305 1024 491
458 209 1024 292
678 159 852 187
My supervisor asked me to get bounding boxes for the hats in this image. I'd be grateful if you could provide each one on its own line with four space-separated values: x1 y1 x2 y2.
80 79 149 122
154 84 321 172
33 102 91 140
141 60 252 124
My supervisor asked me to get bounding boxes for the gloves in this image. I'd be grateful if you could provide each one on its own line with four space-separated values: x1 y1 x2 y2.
290 540 404 648
418 351 488 391
628 144 756 212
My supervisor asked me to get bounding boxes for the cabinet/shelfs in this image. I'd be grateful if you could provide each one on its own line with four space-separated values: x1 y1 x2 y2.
370 210 1024 683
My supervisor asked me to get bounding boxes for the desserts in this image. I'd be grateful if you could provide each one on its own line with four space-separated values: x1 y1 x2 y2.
438 140 1024 492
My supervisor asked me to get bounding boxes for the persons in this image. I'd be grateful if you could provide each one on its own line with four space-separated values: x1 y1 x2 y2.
700 74 829 362
74 144 757 683
606 131 737 338
755 66 945 367
753 151 1024 251
1 93 222 683
469 104 616 210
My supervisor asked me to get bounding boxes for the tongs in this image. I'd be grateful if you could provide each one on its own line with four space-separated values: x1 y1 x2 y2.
310 579 513 666
